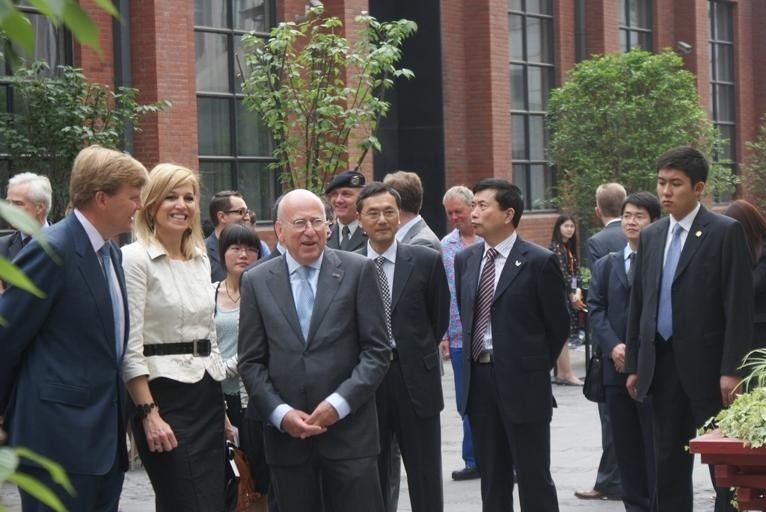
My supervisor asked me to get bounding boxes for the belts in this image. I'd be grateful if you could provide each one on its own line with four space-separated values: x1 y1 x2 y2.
143 339 211 356
473 352 494 365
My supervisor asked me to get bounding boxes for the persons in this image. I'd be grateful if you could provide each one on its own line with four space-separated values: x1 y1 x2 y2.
549 214 586 386
202 171 570 510
576 147 766 512
2 140 238 512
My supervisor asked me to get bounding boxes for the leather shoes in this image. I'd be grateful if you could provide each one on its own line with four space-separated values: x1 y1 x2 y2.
575 490 622 500
452 467 481 480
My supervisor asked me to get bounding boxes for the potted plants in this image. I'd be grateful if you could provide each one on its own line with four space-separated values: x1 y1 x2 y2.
689 386 766 511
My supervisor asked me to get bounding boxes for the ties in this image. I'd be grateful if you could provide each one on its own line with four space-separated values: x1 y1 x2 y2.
626 253 635 284
296 266 315 341
340 227 350 248
472 248 498 362
375 257 392 339
657 224 683 342
100 243 121 357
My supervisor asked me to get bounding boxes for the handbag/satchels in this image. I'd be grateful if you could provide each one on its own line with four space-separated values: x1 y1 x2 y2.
235 450 261 512
583 355 605 402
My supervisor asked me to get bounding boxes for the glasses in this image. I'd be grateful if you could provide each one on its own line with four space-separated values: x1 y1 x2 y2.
225 210 248 215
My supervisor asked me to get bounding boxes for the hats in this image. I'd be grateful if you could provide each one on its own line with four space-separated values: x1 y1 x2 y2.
325 171 365 193
